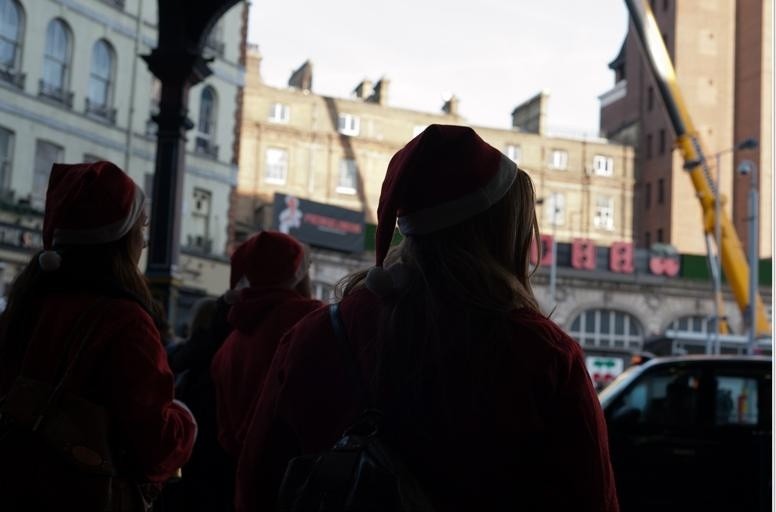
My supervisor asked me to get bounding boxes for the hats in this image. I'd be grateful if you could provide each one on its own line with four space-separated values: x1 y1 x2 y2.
365 124 518 297
223 229 311 305
39 160 146 271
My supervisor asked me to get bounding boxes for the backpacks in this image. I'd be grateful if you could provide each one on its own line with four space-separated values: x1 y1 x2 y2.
277 302 509 512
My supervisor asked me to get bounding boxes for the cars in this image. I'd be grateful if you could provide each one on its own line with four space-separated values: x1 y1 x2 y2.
596 349 771 511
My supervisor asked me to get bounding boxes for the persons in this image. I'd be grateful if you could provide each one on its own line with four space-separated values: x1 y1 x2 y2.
211 231 323 508
167 297 237 510
241 125 617 511
0 160 197 511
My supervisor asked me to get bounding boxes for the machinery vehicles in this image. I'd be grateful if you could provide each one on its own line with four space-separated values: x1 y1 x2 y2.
623 0 773 357
